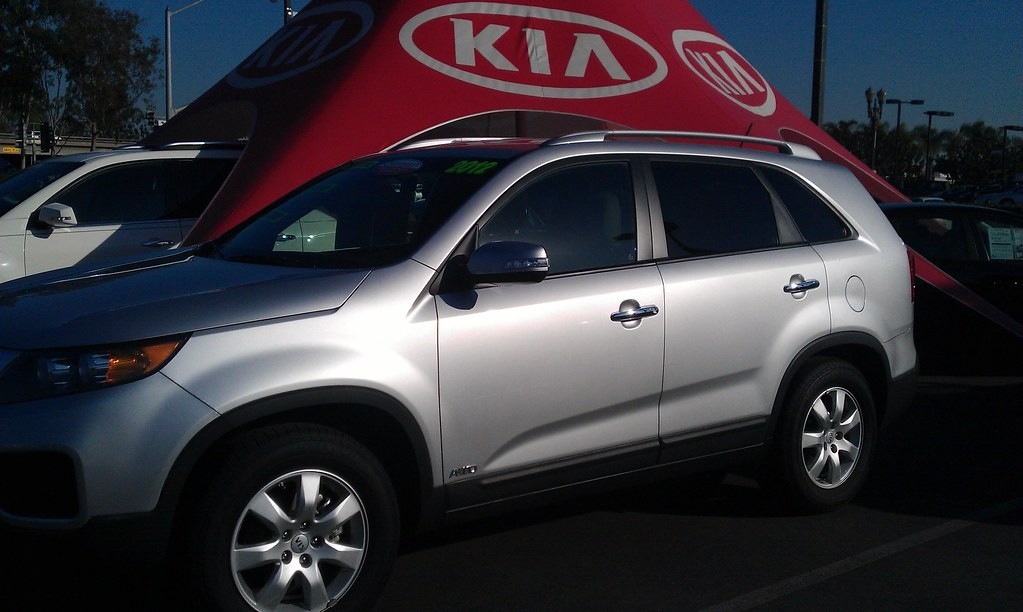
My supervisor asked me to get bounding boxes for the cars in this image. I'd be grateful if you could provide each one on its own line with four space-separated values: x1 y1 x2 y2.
900 180 1023 214
876 200 1023 452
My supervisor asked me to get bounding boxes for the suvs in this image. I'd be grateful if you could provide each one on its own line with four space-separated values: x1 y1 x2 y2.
0 128 922 612
0 137 250 285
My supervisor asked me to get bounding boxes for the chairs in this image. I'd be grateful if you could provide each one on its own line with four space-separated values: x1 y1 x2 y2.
543 188 628 275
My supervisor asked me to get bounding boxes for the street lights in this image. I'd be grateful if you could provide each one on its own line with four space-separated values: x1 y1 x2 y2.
865 85 887 171
923 110 954 193
885 98 925 188
997 125 1023 192
